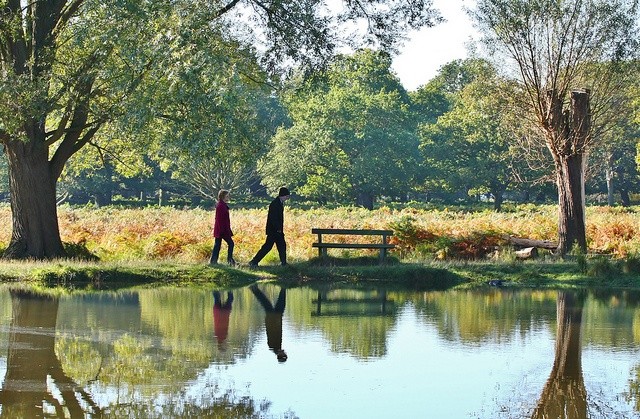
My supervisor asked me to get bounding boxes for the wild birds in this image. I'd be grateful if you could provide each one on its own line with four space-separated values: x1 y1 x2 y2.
485 279 506 287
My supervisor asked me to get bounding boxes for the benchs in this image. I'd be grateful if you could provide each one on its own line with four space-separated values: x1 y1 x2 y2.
310 287 396 319
312 228 395 256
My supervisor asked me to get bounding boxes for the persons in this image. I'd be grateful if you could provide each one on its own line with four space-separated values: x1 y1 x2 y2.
248 187 290 266
210 188 239 266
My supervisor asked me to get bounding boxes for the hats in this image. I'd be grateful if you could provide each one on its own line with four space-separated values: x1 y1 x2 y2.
277 355 287 362
279 188 290 196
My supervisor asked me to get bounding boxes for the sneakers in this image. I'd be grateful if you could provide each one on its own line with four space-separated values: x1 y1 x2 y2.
248 261 261 270
210 261 219 267
228 258 235 266
